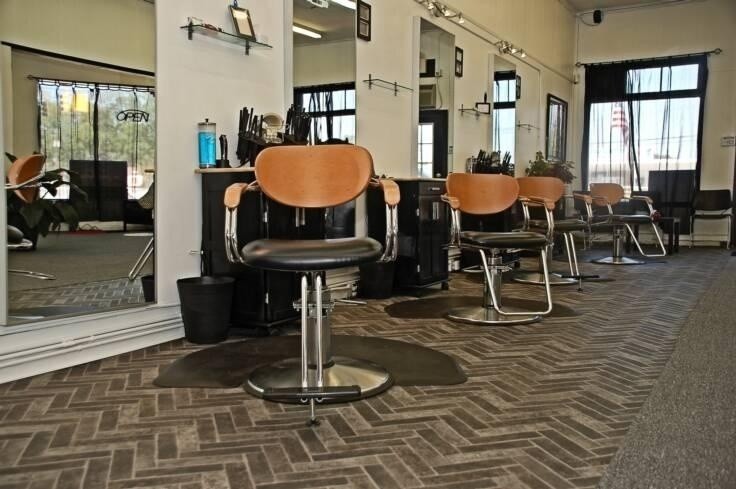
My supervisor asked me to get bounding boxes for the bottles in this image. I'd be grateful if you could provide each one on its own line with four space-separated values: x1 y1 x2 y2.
196 117 217 168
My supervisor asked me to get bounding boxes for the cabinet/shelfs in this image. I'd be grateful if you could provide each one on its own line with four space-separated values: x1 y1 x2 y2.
197 162 327 337
365 177 451 290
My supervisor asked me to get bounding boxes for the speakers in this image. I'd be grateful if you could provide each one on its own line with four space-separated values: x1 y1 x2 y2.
592 9 601 23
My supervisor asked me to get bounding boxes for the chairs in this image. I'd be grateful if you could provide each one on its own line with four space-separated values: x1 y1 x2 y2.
441 172 555 327
512 175 591 293
4 150 155 285
687 188 732 250
574 182 668 265
631 169 700 252
241 145 393 404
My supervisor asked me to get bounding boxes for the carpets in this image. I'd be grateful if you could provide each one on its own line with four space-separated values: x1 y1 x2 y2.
151 333 468 388
596 257 736 489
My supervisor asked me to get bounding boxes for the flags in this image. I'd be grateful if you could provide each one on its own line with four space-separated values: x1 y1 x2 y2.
612 101 629 146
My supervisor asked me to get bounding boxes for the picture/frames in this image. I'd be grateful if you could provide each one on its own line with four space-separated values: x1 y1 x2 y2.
354 1 374 42
453 46 465 79
516 75 523 99
231 4 256 41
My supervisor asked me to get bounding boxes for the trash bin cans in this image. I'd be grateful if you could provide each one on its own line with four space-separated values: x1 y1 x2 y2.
141 275 155 302
176 276 236 344
359 261 395 299
